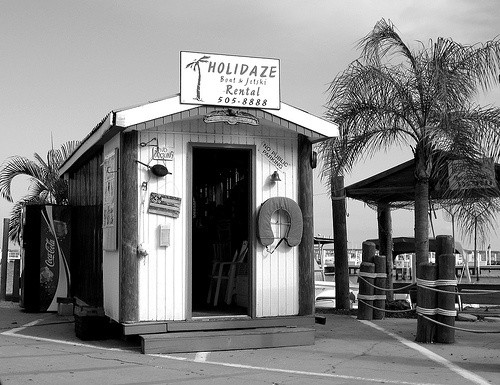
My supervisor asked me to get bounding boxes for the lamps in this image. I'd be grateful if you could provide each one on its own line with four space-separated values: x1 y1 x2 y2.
271 171 281 181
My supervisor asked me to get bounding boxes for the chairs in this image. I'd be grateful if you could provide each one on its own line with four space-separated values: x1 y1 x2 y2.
207 240 249 306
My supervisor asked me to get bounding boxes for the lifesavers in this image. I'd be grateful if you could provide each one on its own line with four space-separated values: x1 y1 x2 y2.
256 197 304 254
